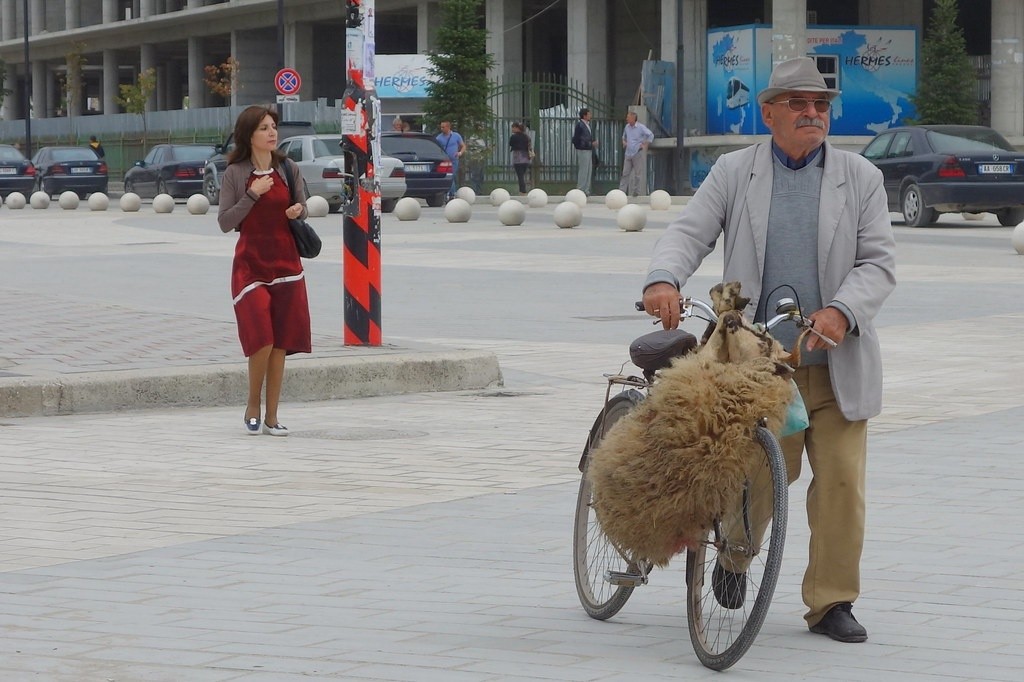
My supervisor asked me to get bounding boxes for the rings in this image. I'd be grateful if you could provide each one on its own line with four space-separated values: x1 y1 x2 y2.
653 309 659 313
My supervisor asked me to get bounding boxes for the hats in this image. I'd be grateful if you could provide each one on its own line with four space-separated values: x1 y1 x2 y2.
757 56 840 107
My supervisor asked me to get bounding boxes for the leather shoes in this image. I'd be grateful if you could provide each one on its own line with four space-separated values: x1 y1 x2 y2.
263 421 289 436
242 405 262 435
808 600 869 642
711 556 751 609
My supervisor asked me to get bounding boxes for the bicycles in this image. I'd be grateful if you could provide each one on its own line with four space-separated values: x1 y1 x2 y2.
573 281 847 672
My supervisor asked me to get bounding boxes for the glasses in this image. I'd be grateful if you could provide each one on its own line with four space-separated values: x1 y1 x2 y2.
769 96 830 115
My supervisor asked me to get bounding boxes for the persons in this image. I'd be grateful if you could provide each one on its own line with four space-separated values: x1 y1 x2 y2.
509 122 533 196
642 57 898 641
618 113 654 196
218 106 313 436
571 109 598 197
392 119 403 130
436 120 466 203
469 124 487 195
89 135 105 159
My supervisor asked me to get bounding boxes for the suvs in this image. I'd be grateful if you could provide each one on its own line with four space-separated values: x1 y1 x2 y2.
382 130 454 207
202 120 317 204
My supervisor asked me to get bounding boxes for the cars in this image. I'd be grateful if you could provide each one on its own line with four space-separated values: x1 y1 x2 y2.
0 144 36 203
33 146 109 200
859 124 1024 228
278 135 408 213
124 143 216 198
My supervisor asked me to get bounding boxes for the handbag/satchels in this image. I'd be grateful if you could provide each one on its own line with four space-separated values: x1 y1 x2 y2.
282 157 321 258
780 377 810 436
528 149 536 159
